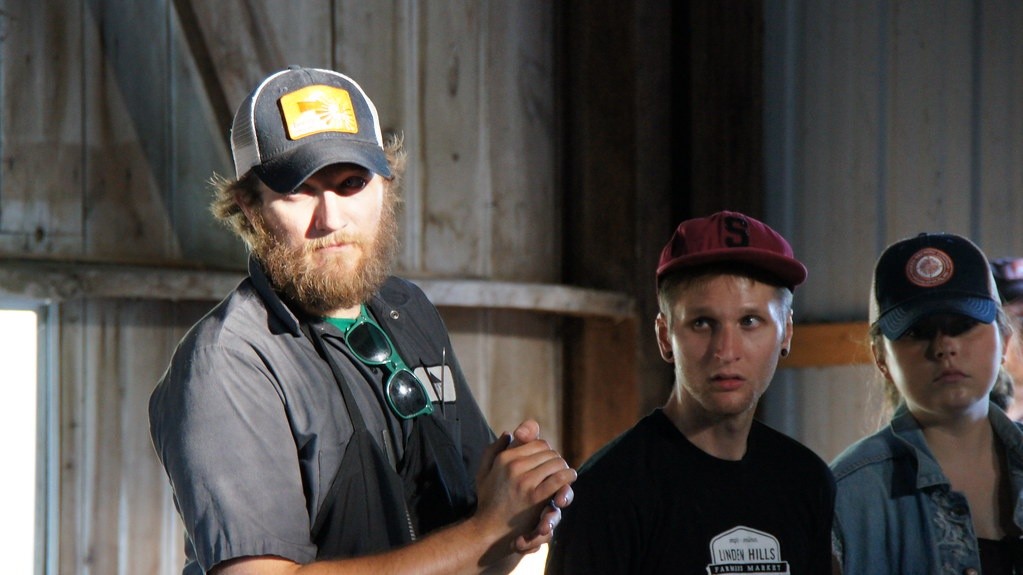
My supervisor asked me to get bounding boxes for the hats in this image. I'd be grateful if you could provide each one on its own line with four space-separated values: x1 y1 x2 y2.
989 257 1023 303
656 210 808 288
229 64 393 195
869 232 1003 342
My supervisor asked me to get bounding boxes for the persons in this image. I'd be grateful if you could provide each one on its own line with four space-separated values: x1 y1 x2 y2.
147 66 577 575
827 233 1023 575
544 210 838 575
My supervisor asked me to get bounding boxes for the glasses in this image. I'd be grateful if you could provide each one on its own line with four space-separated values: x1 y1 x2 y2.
343 316 433 419
